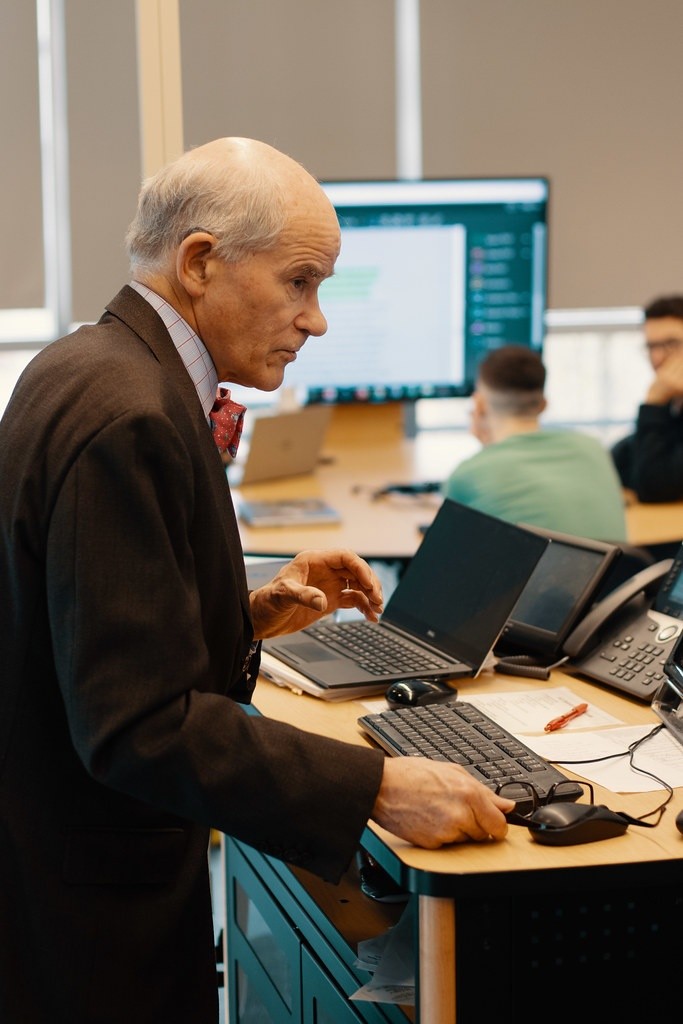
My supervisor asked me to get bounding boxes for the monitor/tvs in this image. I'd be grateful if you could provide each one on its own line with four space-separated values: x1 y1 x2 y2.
500 522 620 649
212 176 551 406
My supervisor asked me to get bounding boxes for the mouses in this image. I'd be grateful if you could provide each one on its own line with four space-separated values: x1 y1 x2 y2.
386 679 458 710
527 802 628 846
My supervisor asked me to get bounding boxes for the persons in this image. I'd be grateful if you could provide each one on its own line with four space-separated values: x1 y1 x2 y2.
608 296 683 503
444 344 630 541
2 133 515 1024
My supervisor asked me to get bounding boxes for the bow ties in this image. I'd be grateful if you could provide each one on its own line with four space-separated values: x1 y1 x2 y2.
209 387 247 460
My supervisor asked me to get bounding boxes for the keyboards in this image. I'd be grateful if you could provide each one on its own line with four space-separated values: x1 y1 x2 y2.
357 700 584 812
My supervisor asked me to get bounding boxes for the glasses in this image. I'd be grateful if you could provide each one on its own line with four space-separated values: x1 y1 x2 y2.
648 336 683 348
494 780 666 832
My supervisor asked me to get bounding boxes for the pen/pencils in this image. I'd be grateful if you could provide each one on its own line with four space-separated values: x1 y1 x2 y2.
543 703 588 733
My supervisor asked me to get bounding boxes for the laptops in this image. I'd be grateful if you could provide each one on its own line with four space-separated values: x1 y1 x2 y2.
257 496 552 691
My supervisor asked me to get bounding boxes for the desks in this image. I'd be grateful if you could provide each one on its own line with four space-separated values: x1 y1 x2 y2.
224 396 683 559
222 666 683 1024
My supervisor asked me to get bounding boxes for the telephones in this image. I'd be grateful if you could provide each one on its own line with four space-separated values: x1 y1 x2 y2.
563 542 683 701
